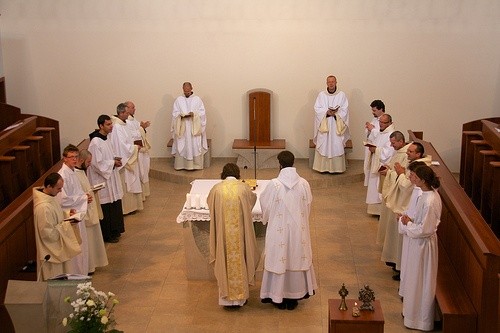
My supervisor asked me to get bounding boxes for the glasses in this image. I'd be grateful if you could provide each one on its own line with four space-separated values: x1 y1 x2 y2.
67 155 79 161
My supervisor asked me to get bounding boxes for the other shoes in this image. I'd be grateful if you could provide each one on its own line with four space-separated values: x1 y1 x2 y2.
286 299 298 310
392 274 400 281
273 301 286 309
106 238 119 243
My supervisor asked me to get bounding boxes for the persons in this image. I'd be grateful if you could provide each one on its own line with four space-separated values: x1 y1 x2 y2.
33 172 82 282
377 131 415 265
55 143 93 275
107 103 143 215
171 82 208 171
207 162 257 309
73 149 109 276
396 161 437 304
382 141 432 280
259 150 318 310
402 164 443 331
363 100 385 186
366 114 395 217
312 75 351 173
123 100 152 202
85 114 125 244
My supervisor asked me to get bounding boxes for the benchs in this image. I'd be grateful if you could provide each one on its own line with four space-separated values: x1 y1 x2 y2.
408 131 500 333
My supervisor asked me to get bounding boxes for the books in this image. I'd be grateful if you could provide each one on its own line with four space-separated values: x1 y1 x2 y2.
378 165 386 171
364 142 376 147
134 140 142 146
328 105 340 111
63 212 86 223
93 182 105 190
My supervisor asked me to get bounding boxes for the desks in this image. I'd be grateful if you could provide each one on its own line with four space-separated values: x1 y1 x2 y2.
176 179 271 282
327 299 385 333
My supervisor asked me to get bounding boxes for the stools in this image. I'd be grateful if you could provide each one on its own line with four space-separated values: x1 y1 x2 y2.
167 139 211 168
309 138 353 167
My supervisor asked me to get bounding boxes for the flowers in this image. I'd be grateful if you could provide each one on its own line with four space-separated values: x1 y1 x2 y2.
62 282 124 333
